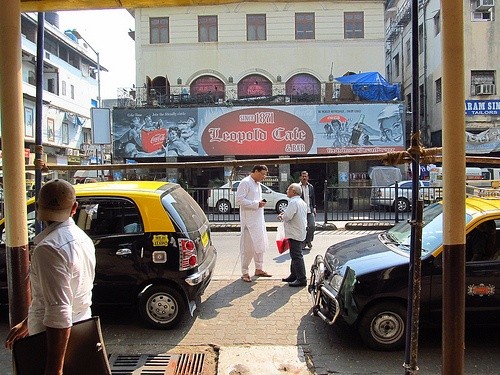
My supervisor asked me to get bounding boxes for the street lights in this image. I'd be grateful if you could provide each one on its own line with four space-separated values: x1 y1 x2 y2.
71 30 101 108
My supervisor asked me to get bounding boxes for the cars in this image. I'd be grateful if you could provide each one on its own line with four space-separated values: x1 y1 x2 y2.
371 180 435 213
308 185 500 351
0 180 217 331
207 180 290 215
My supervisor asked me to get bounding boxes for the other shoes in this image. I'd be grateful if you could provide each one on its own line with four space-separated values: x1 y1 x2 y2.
288 280 305 286
307 242 312 248
282 277 294 282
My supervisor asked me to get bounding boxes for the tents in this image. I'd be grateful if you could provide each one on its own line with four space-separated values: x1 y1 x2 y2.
336 72 399 101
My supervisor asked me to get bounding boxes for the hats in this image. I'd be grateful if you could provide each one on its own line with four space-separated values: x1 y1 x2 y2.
37 179 76 222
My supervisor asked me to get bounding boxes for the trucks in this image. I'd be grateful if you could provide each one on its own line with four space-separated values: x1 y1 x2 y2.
429 167 500 192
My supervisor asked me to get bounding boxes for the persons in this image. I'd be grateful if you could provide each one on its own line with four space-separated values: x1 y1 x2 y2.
379 106 403 146
4 178 96 375
332 120 373 145
236 165 273 282
119 115 198 156
277 183 307 287
300 171 317 249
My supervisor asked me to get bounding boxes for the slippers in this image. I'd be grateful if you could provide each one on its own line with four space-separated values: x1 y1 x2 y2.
241 274 252 282
254 270 272 277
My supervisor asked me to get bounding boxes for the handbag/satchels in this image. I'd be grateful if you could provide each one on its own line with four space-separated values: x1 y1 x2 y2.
276 220 290 254
